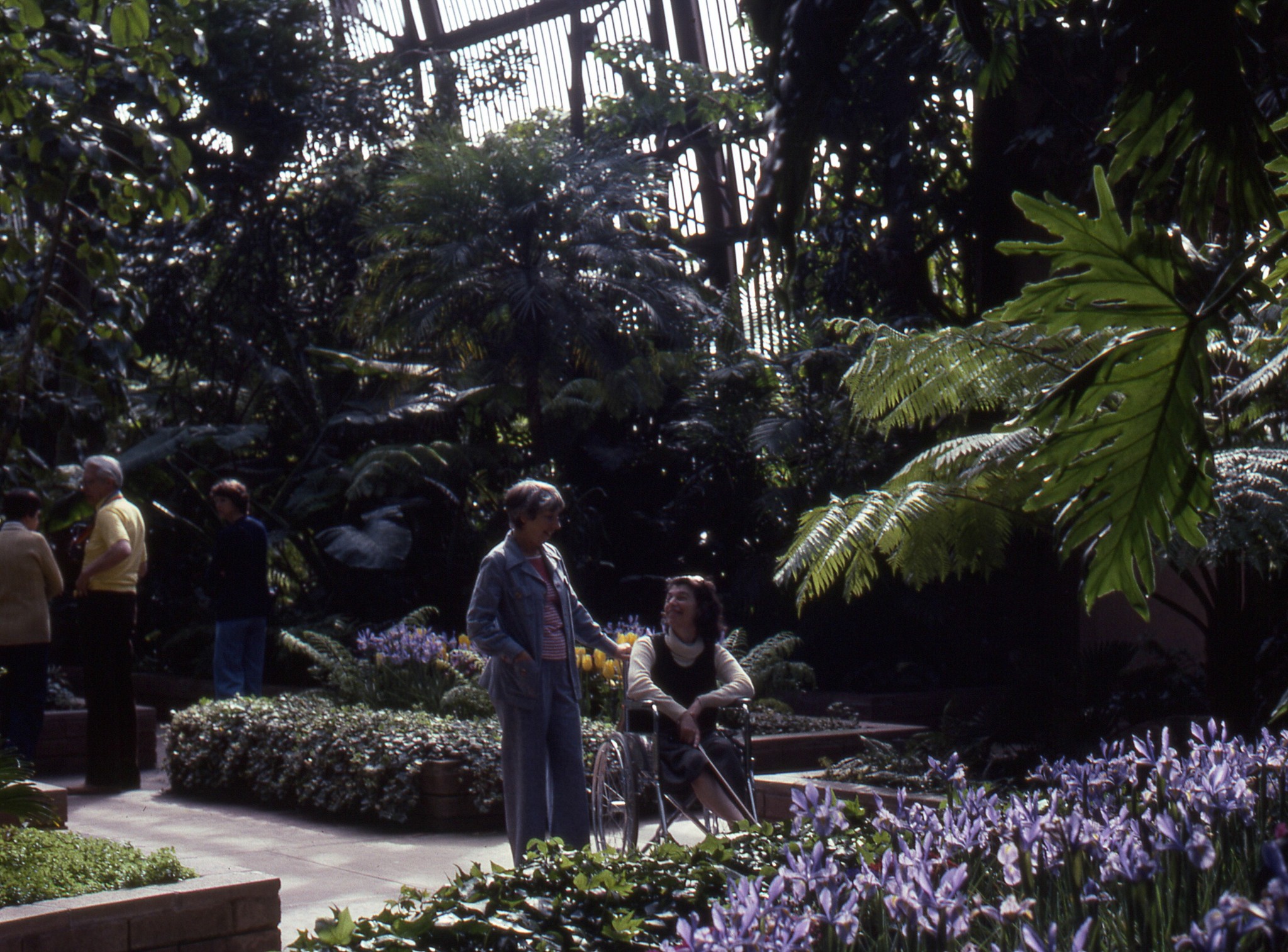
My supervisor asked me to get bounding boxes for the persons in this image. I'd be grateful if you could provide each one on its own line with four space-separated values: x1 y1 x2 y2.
0 488 65 758
626 574 757 833
466 480 633 868
200 479 269 699
65 454 149 795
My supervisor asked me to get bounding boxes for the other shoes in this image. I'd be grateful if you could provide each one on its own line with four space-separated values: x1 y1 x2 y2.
67 780 121 794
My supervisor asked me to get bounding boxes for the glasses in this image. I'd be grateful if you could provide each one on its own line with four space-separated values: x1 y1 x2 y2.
79 479 96 490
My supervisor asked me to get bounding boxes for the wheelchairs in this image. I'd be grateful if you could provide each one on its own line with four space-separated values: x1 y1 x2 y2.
591 653 763 868
